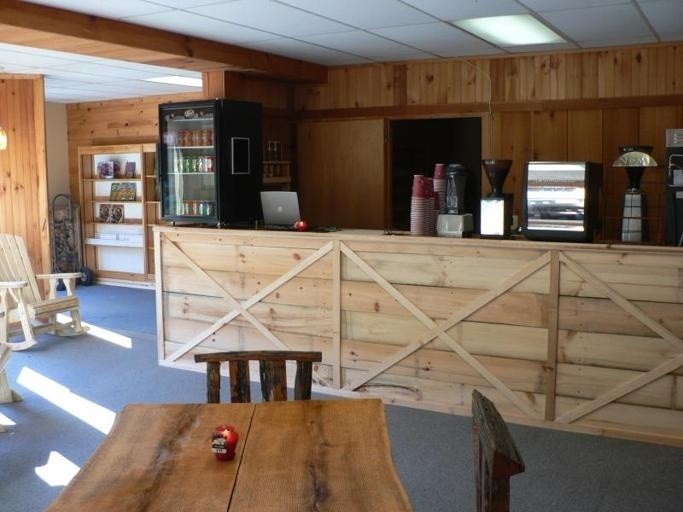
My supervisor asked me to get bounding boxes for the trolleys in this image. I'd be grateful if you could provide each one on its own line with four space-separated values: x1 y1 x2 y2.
52 194 93 291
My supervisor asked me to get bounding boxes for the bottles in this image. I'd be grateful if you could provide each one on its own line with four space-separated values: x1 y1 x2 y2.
206 201 214 216
263 164 291 176
176 130 212 146
175 200 198 216
174 156 215 173
198 200 205 217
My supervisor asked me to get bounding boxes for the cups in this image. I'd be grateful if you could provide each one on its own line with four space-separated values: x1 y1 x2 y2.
409 164 448 236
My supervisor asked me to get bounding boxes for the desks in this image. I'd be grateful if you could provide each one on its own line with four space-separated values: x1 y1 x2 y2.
43 397 412 512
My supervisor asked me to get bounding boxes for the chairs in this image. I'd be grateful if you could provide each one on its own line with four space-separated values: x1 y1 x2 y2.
469 389 526 512
0 233 90 352
196 348 325 404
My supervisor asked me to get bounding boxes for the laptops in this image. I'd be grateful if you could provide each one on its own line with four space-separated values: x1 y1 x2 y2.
261 191 303 230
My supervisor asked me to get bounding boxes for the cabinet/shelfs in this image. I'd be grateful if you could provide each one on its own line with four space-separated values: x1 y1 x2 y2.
151 225 683 449
76 143 144 284
142 145 159 280
521 159 605 241
